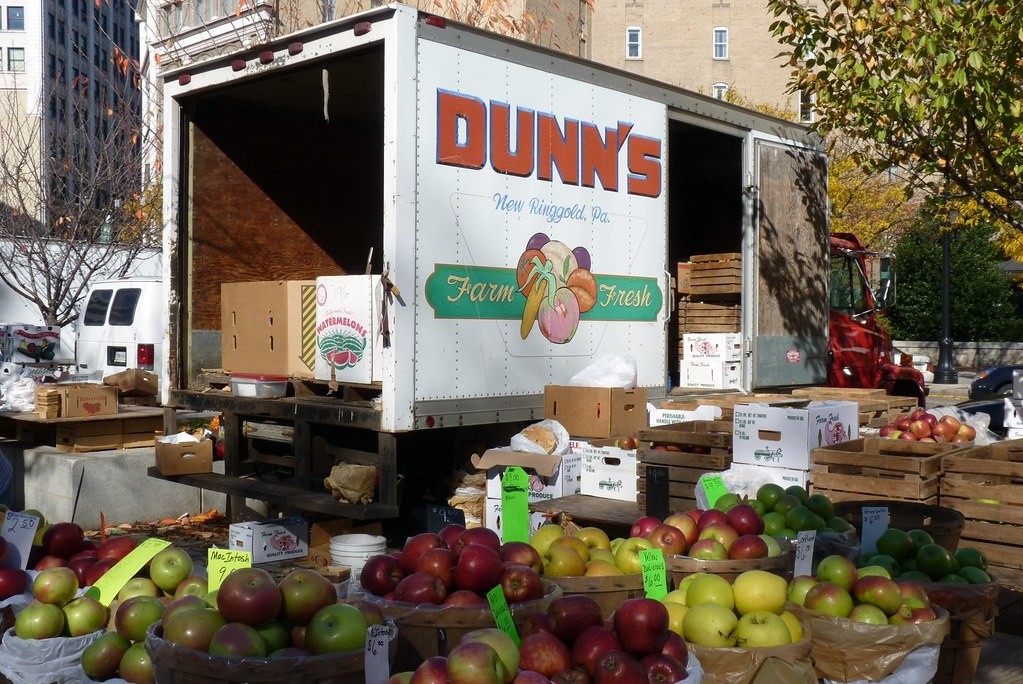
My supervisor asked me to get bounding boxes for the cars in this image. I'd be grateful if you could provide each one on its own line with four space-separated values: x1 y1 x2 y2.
968 364 1023 400
894 346 934 396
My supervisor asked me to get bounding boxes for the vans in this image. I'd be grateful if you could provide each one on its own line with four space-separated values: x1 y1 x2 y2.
75 276 163 407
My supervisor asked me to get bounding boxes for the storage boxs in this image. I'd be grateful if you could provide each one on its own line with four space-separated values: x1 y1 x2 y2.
34 252 1023 592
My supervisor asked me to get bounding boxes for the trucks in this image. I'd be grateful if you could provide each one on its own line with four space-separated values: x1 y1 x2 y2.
146 1 931 518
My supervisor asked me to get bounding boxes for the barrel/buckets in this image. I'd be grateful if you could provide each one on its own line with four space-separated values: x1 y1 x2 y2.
329 533 388 584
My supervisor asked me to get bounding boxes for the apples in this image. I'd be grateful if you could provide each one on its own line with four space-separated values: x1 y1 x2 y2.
879 408 976 444
613 436 732 454
0 483 992 684
